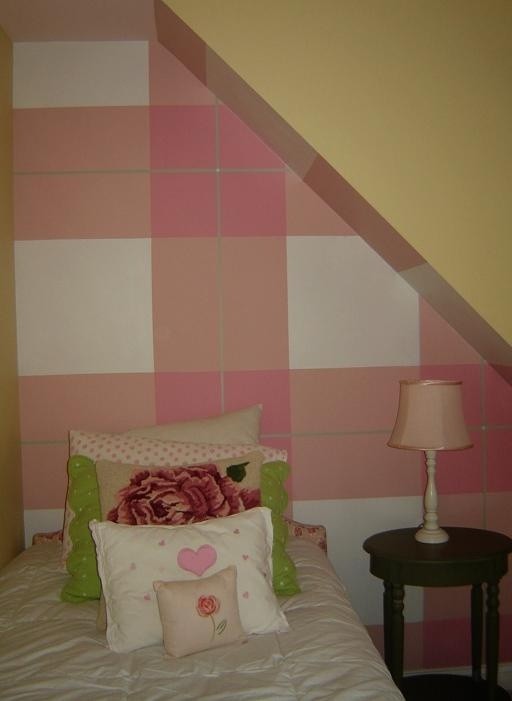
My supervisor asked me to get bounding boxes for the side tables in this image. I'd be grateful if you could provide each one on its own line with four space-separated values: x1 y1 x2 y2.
363 528 511 701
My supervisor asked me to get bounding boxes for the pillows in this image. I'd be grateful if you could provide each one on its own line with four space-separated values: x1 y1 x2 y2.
63 428 289 471
61 453 298 601
92 447 266 529
150 563 250 658
85 505 294 652
120 402 266 448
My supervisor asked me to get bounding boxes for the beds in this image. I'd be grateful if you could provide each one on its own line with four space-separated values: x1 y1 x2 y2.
0 521 408 700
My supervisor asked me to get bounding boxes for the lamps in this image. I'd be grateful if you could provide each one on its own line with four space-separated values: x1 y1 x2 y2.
388 378 474 545
